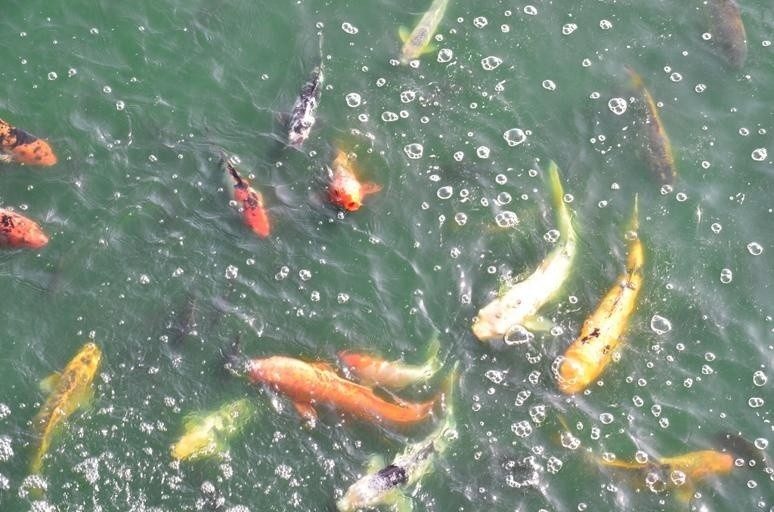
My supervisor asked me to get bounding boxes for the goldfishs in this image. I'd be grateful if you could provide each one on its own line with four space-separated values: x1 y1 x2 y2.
169 327 460 512
